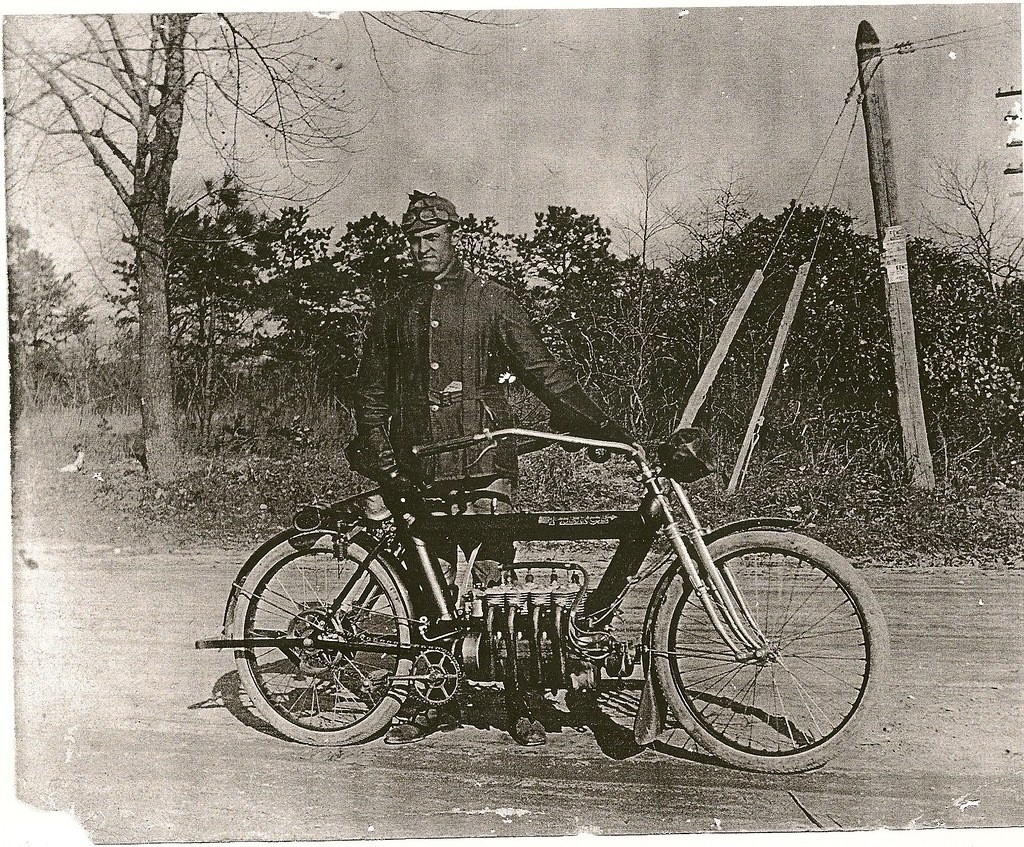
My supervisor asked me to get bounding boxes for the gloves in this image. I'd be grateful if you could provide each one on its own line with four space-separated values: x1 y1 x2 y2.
549 384 631 454
346 425 417 498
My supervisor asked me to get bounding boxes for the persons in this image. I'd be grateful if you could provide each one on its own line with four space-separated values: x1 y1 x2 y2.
344 189 636 746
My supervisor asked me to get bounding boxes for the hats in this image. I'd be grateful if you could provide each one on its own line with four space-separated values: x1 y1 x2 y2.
402 190 457 235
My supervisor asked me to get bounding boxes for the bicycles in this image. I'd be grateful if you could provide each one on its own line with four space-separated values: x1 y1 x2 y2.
194 421 892 777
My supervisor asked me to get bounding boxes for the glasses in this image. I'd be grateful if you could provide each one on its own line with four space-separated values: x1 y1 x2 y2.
401 206 439 227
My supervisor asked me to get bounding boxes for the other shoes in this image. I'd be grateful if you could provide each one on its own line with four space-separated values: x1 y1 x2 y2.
385 707 457 744
505 705 545 746
